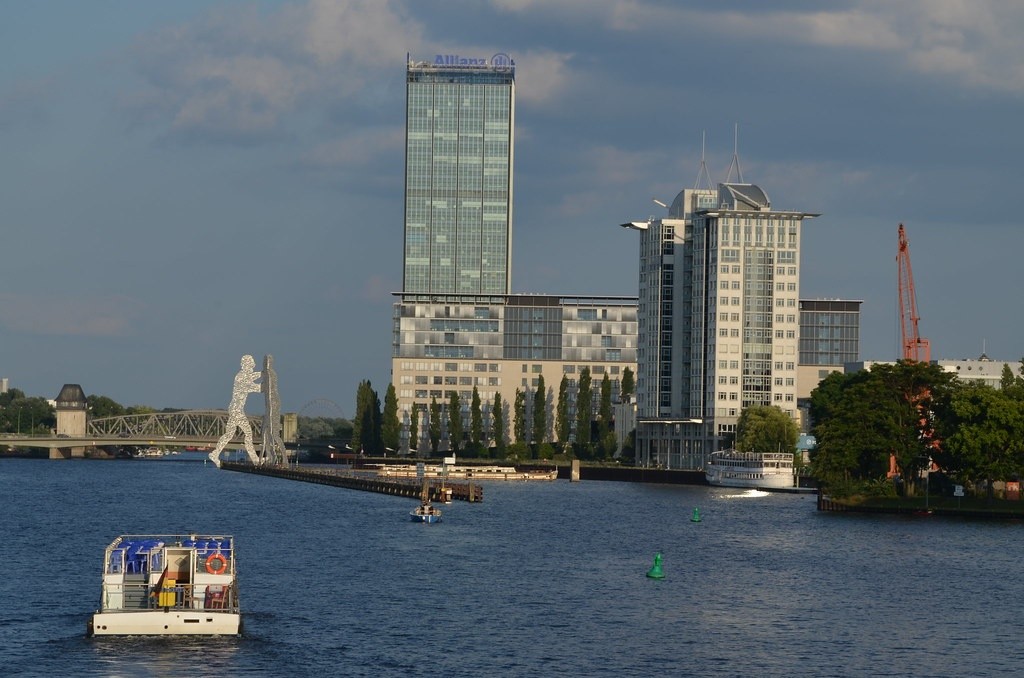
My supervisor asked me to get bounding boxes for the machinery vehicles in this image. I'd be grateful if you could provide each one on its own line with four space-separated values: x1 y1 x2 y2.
888 223 944 476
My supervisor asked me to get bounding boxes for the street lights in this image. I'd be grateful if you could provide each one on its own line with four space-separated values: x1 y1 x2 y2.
108 406 114 433
17 407 23 435
30 406 34 438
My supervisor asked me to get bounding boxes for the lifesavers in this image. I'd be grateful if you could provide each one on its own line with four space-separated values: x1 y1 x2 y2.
206 555 227 575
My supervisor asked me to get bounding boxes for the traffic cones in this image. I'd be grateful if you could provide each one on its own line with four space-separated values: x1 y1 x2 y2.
646 551 665 578
690 506 701 522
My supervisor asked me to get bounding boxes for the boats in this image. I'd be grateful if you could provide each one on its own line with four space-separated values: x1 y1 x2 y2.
185 446 210 451
705 440 797 487
131 446 181 458
409 492 442 524
